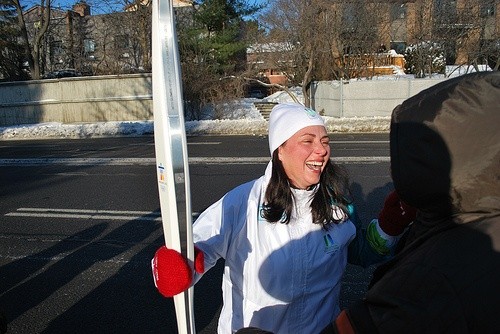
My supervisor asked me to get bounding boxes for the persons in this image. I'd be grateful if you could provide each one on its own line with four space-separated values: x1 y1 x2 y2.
315 70 500 334
150 102 414 333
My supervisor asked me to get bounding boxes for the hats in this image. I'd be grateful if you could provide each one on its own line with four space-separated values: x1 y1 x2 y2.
269 103 328 159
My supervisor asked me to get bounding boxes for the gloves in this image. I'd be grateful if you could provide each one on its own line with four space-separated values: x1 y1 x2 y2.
378 191 417 236
151 243 205 299
